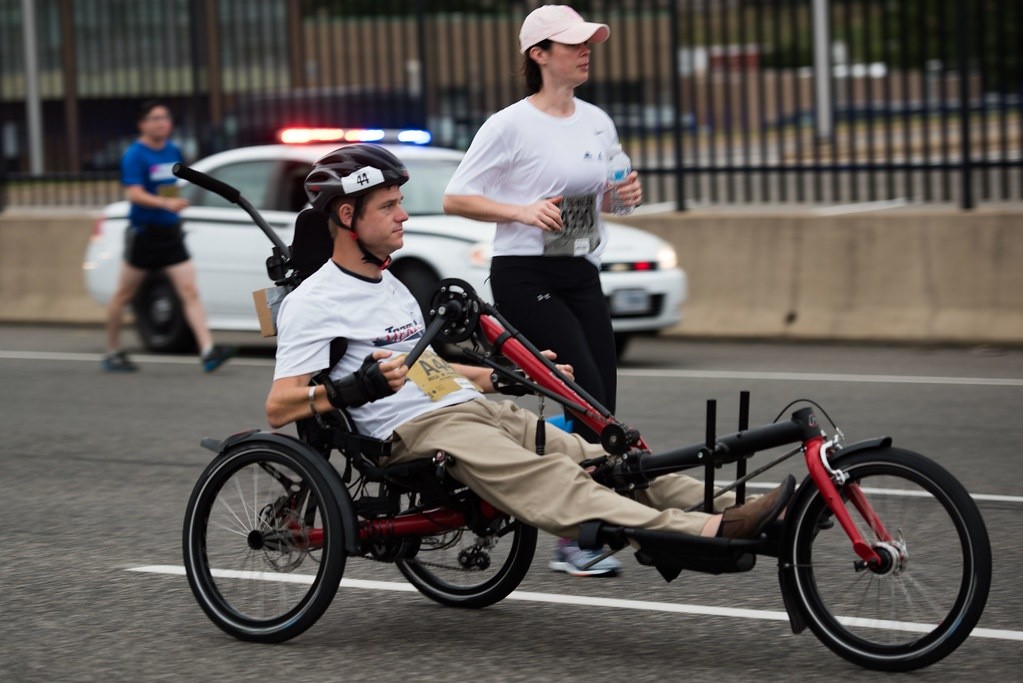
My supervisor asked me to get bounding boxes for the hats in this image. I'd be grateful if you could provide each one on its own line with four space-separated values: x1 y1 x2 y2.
519 4 609 56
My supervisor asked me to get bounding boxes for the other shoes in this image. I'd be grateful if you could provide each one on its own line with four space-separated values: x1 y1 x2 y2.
204 344 239 371
720 473 796 547
100 351 136 371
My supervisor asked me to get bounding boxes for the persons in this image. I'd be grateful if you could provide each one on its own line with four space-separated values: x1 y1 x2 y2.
269 144 799 545
103 105 239 372
441 6 642 576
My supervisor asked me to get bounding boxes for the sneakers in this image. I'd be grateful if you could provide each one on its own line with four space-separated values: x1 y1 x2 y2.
547 546 621 577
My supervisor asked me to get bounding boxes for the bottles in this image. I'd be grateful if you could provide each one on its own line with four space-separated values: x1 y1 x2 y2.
606 143 635 217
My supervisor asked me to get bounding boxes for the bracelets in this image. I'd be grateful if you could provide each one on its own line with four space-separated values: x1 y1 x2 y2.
309 386 319 415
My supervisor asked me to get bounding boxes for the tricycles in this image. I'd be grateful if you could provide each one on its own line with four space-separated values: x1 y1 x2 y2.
162 163 992 673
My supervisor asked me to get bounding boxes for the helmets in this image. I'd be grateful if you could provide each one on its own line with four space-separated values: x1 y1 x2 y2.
303 143 409 212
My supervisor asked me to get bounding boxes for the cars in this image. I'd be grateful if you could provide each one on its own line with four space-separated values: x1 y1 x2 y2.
84 125 687 360
